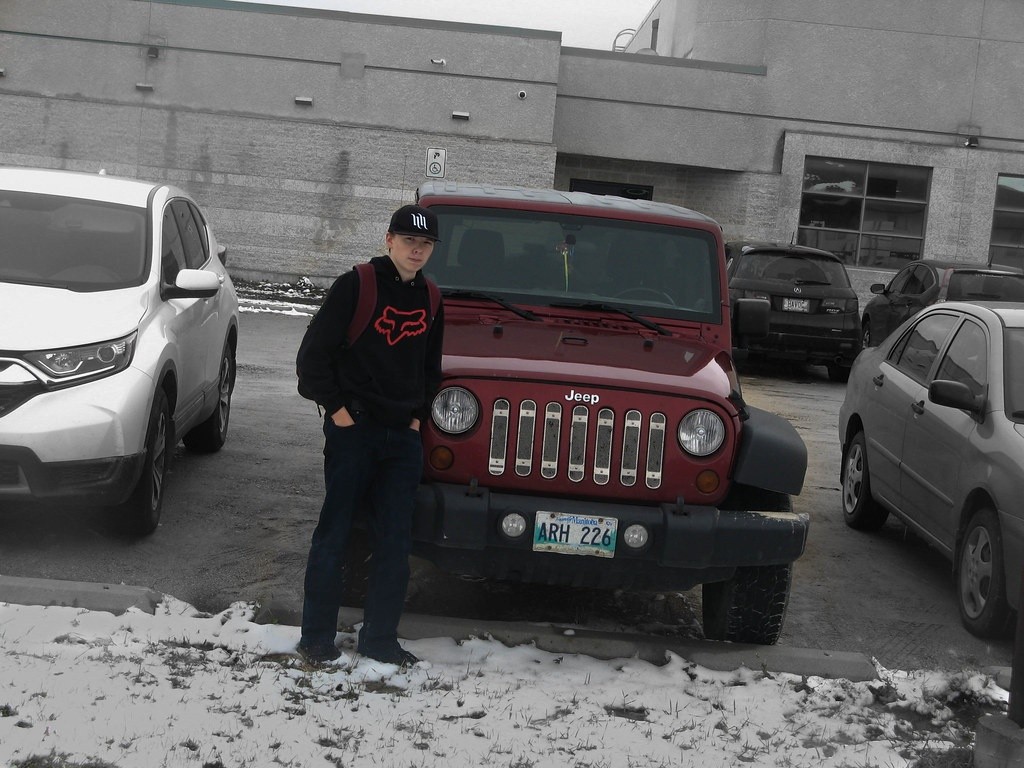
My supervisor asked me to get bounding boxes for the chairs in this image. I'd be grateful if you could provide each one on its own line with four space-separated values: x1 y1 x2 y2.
592 239 667 300
446 227 508 289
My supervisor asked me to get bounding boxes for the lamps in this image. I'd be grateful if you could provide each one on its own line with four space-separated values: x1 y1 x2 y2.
135 82 155 92
452 111 471 120
146 48 158 59
0 68 6 77
294 96 313 106
965 135 978 149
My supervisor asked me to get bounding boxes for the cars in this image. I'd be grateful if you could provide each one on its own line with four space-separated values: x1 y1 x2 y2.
836 299 1024 647
0 166 240 538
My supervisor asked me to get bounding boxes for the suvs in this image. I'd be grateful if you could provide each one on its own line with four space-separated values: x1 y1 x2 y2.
861 259 1023 354
725 237 862 385
409 177 811 647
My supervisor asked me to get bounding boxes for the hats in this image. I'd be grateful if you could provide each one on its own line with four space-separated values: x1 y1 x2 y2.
388 203 441 243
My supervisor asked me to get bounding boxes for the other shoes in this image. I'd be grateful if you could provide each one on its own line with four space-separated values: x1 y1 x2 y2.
297 639 354 673
360 644 418 668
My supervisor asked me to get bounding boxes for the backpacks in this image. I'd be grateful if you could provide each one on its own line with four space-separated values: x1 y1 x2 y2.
296 264 440 402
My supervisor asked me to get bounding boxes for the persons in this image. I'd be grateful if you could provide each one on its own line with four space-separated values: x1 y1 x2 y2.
295 204 446 673
